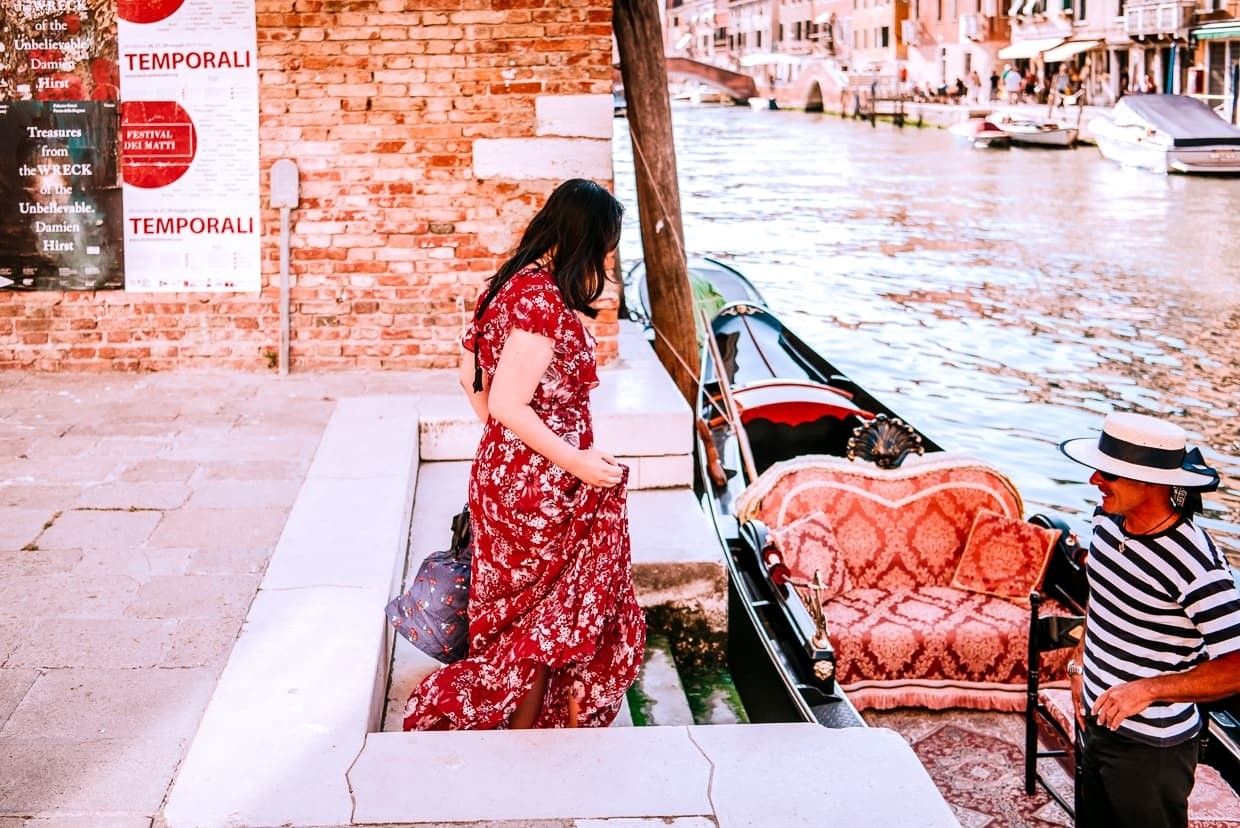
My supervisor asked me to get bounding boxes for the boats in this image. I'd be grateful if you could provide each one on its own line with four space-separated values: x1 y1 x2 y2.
974 115 1078 149
695 299 1239 828
623 247 770 350
1087 95 1240 174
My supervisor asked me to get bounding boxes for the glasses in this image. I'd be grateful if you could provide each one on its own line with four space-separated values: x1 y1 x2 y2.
1097 469 1124 483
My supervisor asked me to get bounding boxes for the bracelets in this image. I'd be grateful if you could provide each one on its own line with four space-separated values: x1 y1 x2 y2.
1067 660 1082 676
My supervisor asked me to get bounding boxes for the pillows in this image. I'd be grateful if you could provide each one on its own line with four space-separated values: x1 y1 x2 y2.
953 510 1064 604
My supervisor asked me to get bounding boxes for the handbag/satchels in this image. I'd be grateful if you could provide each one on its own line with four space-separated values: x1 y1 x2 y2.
381 505 484 664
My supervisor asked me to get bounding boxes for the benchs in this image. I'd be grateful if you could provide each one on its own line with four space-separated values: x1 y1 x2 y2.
730 441 1076 708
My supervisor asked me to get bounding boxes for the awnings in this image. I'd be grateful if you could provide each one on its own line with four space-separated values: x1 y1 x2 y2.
1043 40 1104 62
1192 21 1240 40
998 40 1065 59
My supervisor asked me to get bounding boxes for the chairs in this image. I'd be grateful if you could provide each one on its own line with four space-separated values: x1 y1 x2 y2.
1020 581 1085 828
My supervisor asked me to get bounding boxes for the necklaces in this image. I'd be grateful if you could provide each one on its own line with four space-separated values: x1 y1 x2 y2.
1119 509 1176 552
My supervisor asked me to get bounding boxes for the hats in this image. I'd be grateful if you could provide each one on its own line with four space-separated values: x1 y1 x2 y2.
1058 411 1220 494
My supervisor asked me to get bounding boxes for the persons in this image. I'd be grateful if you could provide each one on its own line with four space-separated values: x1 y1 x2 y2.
404 179 646 730
990 64 1081 105
913 70 981 103
1059 412 1240 828
1123 66 1156 94
